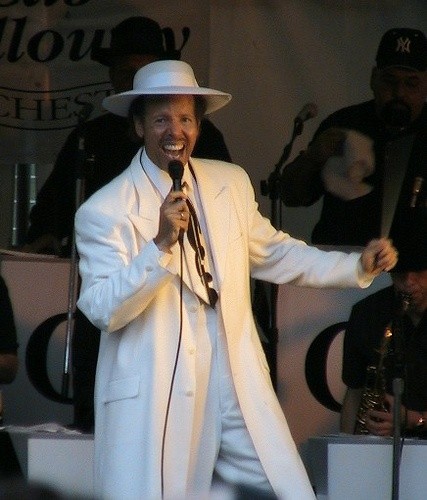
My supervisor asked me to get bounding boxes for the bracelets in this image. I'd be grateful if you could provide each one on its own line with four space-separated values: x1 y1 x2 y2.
404 408 408 434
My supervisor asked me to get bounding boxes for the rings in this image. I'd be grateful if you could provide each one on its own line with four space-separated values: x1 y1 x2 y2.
180 211 184 220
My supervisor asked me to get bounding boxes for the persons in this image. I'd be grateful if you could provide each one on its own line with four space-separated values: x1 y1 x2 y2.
5 18 232 434
340 207 427 441
75 60 397 500
281 29 427 246
0 277 24 481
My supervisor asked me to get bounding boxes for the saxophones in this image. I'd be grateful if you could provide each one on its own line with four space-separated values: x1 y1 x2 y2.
351 290 418 436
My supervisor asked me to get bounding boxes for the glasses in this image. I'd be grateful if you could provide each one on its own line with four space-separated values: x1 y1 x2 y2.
372 73 427 94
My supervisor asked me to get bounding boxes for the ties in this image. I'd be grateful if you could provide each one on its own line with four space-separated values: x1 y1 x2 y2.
173 186 219 310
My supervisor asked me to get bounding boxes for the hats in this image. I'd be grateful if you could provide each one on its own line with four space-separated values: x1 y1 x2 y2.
102 59 232 117
375 27 427 73
90 16 181 66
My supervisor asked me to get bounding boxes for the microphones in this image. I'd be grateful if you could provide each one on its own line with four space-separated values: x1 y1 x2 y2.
168 159 185 243
294 102 319 128
392 378 404 395
